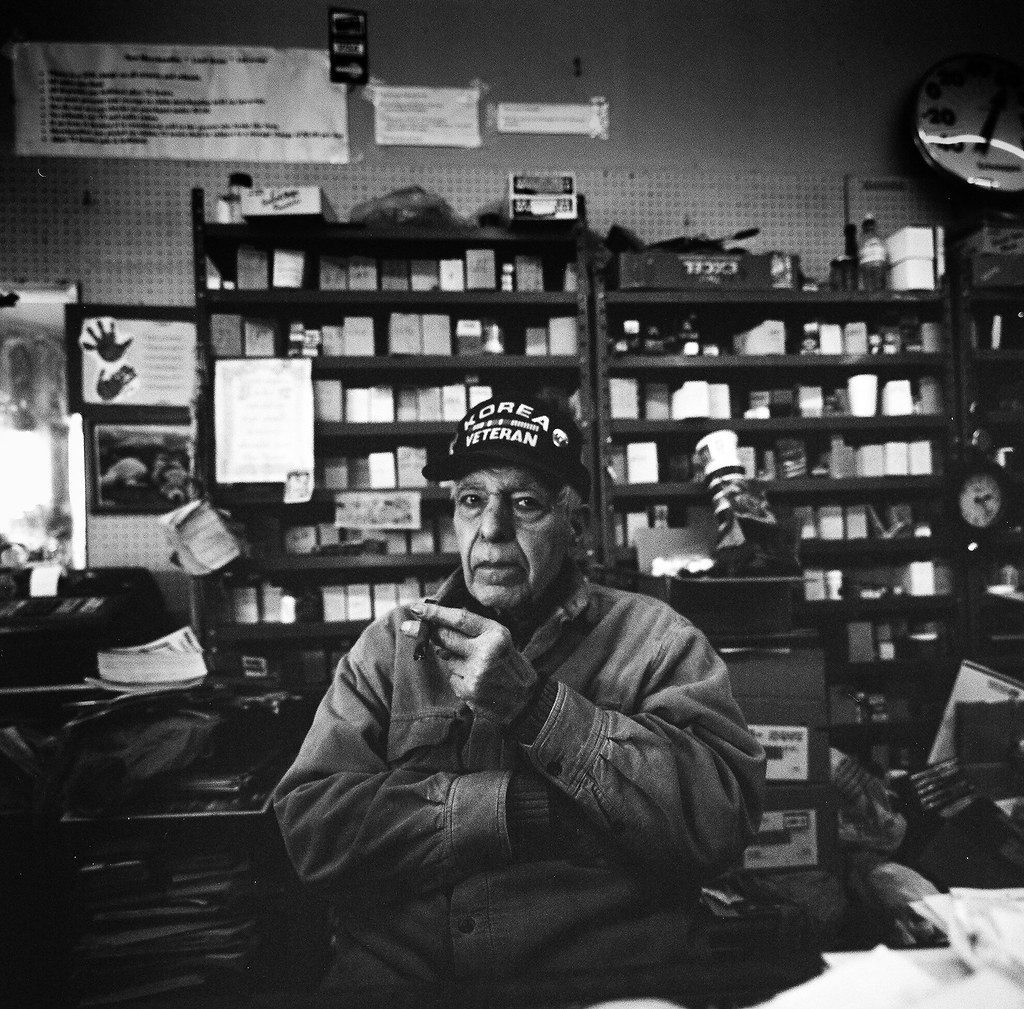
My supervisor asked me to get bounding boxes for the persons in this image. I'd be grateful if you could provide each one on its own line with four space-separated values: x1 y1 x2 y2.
274 398 767 991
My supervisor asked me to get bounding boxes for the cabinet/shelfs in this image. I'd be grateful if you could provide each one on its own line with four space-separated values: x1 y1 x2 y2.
193 185 1022 751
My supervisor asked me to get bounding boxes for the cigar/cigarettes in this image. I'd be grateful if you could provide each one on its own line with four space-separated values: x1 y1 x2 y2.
413 599 439 661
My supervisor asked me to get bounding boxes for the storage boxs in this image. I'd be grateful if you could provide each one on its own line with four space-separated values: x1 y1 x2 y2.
628 568 839 875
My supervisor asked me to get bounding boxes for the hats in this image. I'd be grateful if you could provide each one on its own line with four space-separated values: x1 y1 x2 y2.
421 395 582 493
